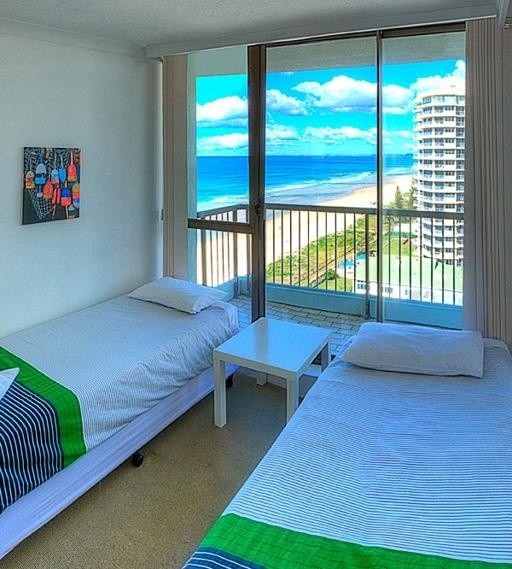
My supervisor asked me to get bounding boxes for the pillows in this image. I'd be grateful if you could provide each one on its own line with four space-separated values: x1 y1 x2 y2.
129 276 228 315
340 322 484 378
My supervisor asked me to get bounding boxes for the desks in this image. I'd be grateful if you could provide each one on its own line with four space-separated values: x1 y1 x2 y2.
212 317 333 428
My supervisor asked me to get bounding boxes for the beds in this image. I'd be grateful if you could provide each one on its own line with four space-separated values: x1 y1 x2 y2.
0 292 240 561
183 334 512 569
